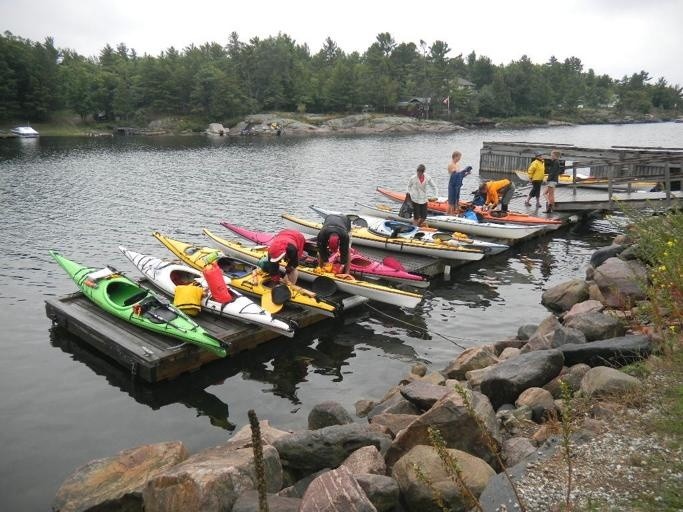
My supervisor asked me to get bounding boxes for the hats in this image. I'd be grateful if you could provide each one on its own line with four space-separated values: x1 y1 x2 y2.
269 241 288 263
535 151 544 158
328 233 339 253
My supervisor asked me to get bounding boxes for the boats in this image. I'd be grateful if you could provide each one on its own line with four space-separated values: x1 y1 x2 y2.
220 222 431 289
119 245 294 338
280 214 485 261
50 249 226 358
11 120 39 138
309 205 511 256
203 228 423 308
355 202 547 239
377 187 562 230
152 231 337 318
512 170 658 192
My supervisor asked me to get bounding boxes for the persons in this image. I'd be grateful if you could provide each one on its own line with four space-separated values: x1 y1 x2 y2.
316 213 352 273
268 229 305 285
404 164 438 226
478 178 515 212
524 151 546 207
445 151 473 215
542 152 561 213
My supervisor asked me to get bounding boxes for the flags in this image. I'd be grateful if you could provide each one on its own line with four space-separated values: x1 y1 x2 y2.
440 97 448 105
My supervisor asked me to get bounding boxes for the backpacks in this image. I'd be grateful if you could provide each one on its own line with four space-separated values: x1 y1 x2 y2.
398 192 415 219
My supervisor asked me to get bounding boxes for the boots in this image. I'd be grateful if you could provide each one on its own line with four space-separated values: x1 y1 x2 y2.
501 204 508 213
542 204 553 213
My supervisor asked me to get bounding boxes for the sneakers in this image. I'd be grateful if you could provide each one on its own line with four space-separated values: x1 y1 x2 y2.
525 201 531 207
536 202 542 208
